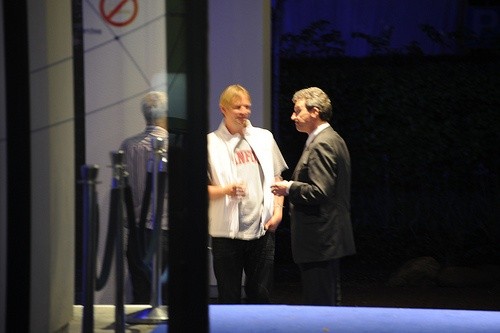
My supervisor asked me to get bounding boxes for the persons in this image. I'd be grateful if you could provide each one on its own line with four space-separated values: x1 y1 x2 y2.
114 86 177 308
268 84 354 305
202 82 286 305
